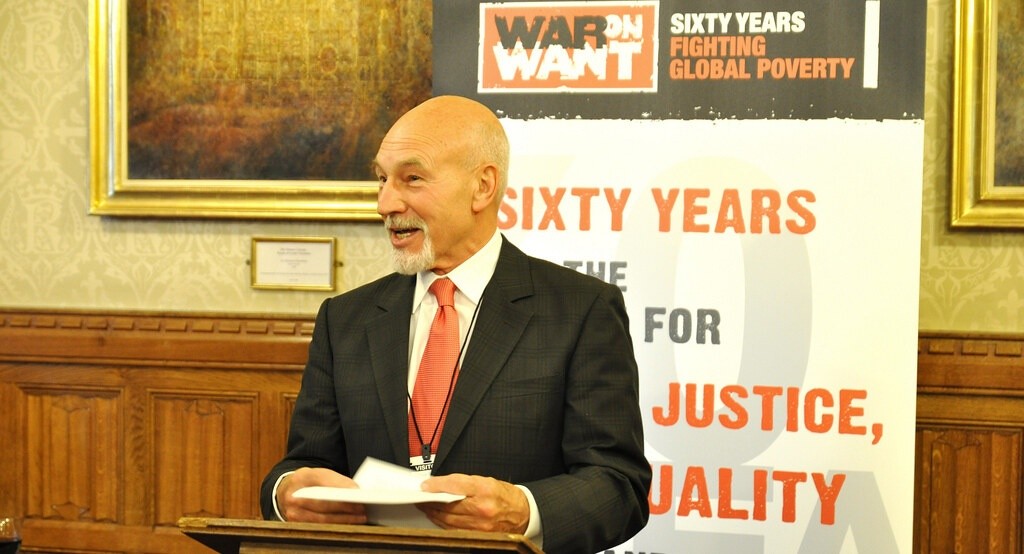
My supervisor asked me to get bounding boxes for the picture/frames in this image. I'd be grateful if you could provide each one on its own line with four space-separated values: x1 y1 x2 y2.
86 1 438 224
950 0 1023 227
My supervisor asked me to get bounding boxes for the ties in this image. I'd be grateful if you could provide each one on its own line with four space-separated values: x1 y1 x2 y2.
408 278 459 458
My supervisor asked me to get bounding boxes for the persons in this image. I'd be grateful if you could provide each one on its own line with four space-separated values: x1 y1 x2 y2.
259 95 652 554
